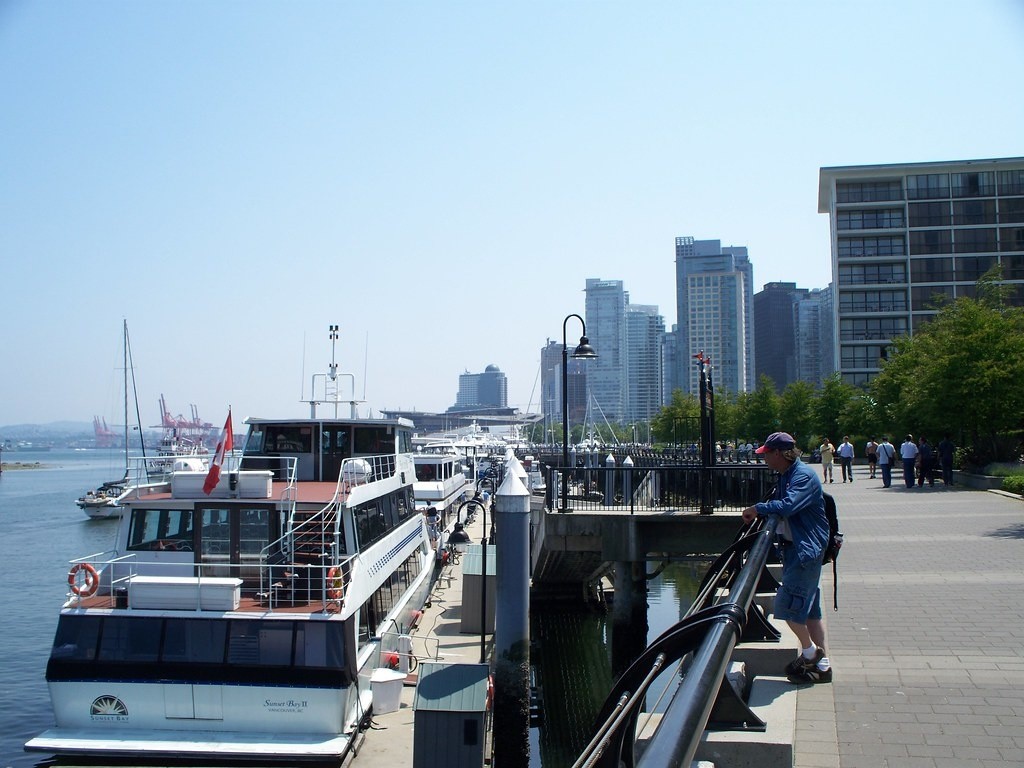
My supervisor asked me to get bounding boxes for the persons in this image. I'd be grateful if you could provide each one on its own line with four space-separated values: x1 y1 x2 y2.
865 436 879 479
425 501 441 542
820 438 835 483
810 446 820 463
899 434 919 489
938 435 957 486
877 437 897 489
917 436 934 488
483 490 489 508
837 436 854 483
743 432 832 685
663 440 762 464
460 492 468 504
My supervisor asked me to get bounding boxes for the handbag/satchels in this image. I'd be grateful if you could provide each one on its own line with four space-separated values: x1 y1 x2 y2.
888 457 895 467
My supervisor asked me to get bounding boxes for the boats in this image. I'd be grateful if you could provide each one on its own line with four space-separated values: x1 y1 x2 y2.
1 320 477 768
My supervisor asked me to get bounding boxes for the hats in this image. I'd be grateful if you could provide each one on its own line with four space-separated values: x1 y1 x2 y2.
754 432 794 454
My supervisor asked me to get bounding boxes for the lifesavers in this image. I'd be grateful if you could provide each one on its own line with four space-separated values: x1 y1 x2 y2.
67 564 98 597
486 676 495 709
327 568 344 600
154 542 178 551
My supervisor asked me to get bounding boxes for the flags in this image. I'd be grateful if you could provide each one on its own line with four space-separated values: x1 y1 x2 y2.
202 410 234 496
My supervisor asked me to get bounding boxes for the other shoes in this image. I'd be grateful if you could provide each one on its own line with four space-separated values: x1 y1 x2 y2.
824 480 827 483
830 479 834 483
870 476 873 479
850 478 853 482
929 483 934 487
843 480 846 483
917 484 922 488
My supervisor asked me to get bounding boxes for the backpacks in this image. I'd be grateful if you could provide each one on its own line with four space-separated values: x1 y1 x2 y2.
821 491 843 565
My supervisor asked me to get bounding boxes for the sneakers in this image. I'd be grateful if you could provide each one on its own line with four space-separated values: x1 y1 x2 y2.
785 646 825 676
787 664 832 684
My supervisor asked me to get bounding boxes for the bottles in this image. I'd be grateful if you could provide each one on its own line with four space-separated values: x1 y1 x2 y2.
829 532 843 561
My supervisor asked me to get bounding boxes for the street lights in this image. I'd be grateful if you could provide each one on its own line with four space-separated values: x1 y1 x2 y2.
445 500 487 663
560 313 599 513
485 460 503 494
468 478 496 546
650 428 654 445
632 426 634 445
